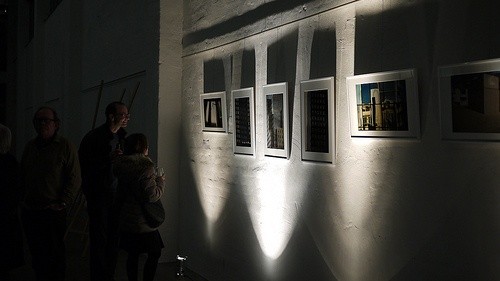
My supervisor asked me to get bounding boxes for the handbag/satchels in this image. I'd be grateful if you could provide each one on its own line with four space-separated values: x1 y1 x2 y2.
140 200 165 228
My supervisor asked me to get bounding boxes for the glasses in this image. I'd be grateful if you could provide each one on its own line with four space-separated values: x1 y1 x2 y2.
32 119 56 125
116 112 131 118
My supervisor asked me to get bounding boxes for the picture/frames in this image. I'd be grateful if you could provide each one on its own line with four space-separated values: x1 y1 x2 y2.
437 58 500 142
353 68 422 139
199 90 228 133
299 76 334 164
264 81 290 158
230 86 255 155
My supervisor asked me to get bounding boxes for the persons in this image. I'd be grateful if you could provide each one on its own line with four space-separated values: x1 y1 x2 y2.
0 125 27 281
18 106 81 281
78 101 131 281
111 133 165 281
